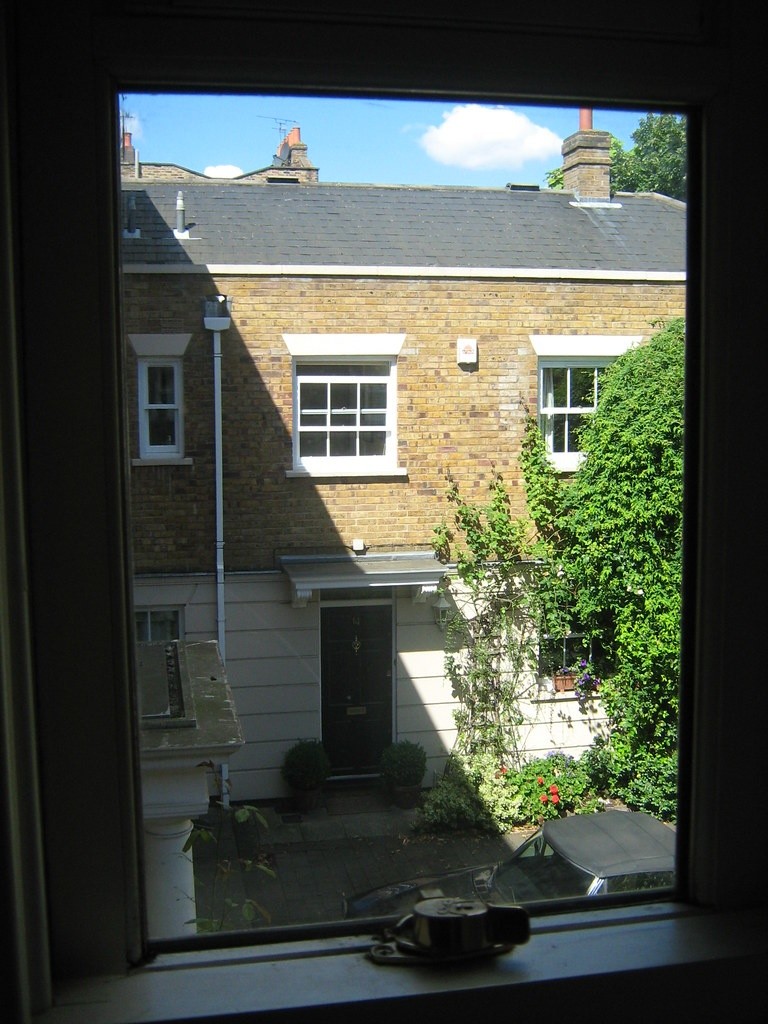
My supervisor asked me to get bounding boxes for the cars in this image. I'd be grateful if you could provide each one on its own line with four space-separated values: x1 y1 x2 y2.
339 810 675 919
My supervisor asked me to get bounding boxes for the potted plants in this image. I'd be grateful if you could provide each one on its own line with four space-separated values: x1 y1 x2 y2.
381 740 427 809
281 735 327 812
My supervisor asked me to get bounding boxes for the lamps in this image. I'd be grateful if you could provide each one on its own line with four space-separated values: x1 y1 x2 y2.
432 593 452 631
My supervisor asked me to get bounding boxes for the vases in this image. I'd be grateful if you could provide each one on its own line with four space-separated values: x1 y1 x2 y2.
555 676 578 691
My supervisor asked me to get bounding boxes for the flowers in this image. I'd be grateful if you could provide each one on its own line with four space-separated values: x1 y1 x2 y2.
555 658 597 702
